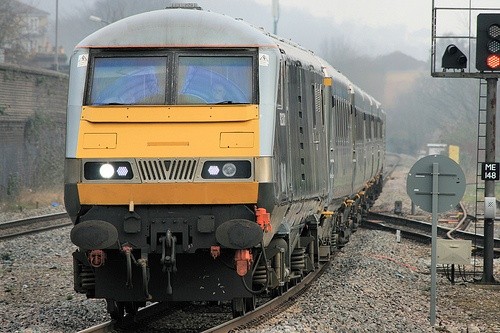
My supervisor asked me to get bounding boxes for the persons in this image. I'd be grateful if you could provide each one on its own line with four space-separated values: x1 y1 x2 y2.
211 81 227 102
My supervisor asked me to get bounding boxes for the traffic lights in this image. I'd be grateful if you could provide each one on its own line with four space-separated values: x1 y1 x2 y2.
477 12 500 72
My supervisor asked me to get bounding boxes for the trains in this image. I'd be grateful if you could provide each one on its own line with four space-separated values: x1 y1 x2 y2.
60 3 387 328
